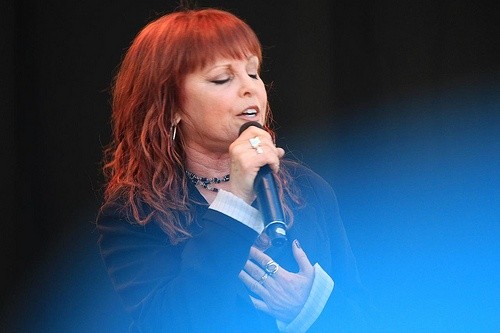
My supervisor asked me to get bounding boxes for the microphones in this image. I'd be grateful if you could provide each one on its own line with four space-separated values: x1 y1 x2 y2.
237 121 288 249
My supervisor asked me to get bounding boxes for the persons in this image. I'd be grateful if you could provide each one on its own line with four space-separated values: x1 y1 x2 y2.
94 7 367 332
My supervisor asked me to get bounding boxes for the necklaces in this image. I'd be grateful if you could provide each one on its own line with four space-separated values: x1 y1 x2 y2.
183 168 232 193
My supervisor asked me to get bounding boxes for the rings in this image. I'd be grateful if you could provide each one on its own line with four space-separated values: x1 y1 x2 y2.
256 147 263 154
265 261 280 276
249 137 262 148
259 274 268 283
